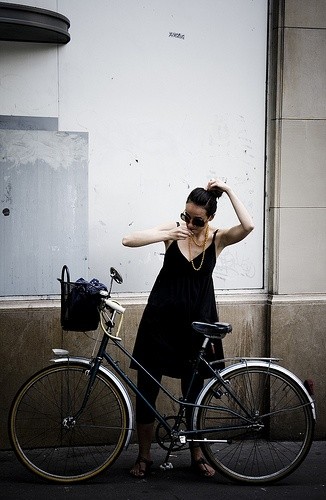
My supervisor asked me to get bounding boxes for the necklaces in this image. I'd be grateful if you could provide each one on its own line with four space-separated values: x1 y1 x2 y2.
186 225 209 271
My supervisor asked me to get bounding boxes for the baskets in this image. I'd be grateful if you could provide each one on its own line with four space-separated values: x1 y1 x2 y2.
61 264 101 332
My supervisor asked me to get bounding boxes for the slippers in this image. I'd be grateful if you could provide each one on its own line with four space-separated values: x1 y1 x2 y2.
190 457 217 480
130 455 153 480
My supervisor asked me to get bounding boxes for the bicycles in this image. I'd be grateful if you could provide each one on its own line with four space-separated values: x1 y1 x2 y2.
9 265 317 485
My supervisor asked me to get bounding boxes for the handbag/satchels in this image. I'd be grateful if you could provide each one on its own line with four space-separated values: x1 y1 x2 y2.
72 278 108 309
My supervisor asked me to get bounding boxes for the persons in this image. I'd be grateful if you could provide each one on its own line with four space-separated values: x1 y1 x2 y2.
121 179 254 479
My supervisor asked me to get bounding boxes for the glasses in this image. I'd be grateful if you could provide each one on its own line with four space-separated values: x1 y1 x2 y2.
180 209 211 227
